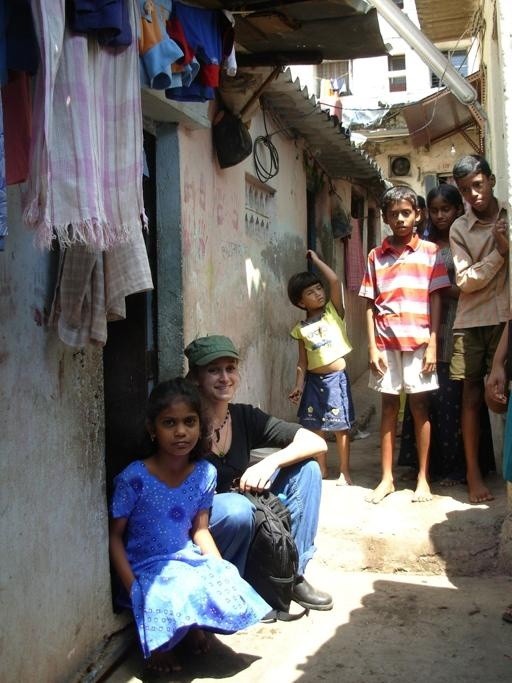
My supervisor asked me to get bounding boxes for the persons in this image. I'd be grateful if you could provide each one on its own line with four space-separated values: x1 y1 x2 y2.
442 153 511 504
286 250 359 486
180 333 337 610
482 316 511 626
395 181 482 491
359 186 448 507
106 376 257 676
415 194 431 241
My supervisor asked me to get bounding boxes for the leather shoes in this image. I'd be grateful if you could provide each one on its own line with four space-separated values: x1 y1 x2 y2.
290 576 333 610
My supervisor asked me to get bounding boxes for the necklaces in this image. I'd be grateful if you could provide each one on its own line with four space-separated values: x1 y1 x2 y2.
210 420 229 457
209 407 232 442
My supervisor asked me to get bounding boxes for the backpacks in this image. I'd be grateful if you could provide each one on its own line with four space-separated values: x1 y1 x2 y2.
228 477 300 612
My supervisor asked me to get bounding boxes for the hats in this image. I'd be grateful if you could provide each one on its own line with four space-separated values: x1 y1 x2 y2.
184 334 244 366
